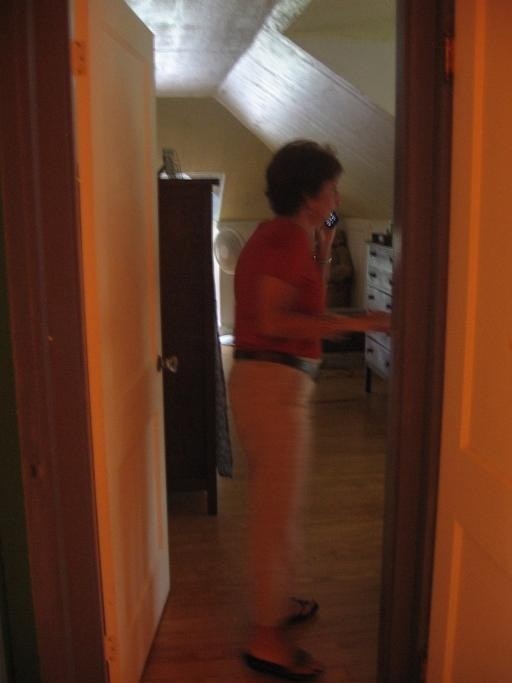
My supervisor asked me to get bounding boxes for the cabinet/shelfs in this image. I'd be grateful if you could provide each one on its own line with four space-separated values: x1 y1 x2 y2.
158 176 220 516
362 239 394 394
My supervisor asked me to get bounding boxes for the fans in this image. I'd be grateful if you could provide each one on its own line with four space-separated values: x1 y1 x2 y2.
213 226 246 346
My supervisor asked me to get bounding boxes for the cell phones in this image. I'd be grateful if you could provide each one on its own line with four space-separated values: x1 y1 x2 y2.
325 210 336 229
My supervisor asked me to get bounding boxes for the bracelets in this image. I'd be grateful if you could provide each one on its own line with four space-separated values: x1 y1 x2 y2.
313 254 333 264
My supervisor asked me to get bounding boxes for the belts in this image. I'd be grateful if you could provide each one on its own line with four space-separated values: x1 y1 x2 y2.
233 351 321 382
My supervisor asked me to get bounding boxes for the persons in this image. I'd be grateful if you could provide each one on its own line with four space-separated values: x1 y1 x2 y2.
225 138 391 683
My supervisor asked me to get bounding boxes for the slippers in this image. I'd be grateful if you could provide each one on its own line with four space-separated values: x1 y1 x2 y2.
245 648 321 682
287 599 319 622
